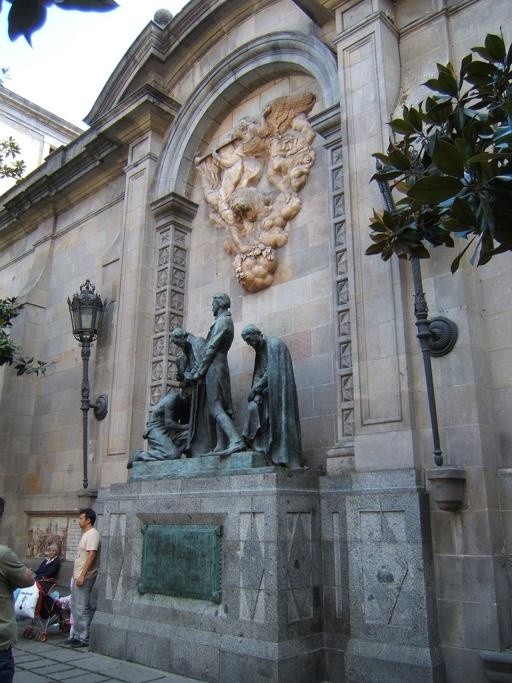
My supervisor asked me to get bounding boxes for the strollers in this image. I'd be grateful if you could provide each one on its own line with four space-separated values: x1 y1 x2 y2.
22 576 71 642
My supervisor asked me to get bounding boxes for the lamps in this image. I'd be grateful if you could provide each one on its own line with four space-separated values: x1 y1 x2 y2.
65 277 108 489
374 137 460 468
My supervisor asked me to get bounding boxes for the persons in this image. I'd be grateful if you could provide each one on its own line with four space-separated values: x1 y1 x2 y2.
67 576 75 640
190 292 247 457
0 497 36 683
170 327 217 457
64 508 101 648
49 590 71 609
240 323 304 469
126 385 192 469
34 544 61 592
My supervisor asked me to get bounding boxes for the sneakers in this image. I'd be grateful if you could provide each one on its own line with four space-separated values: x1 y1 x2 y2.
65 638 89 647
